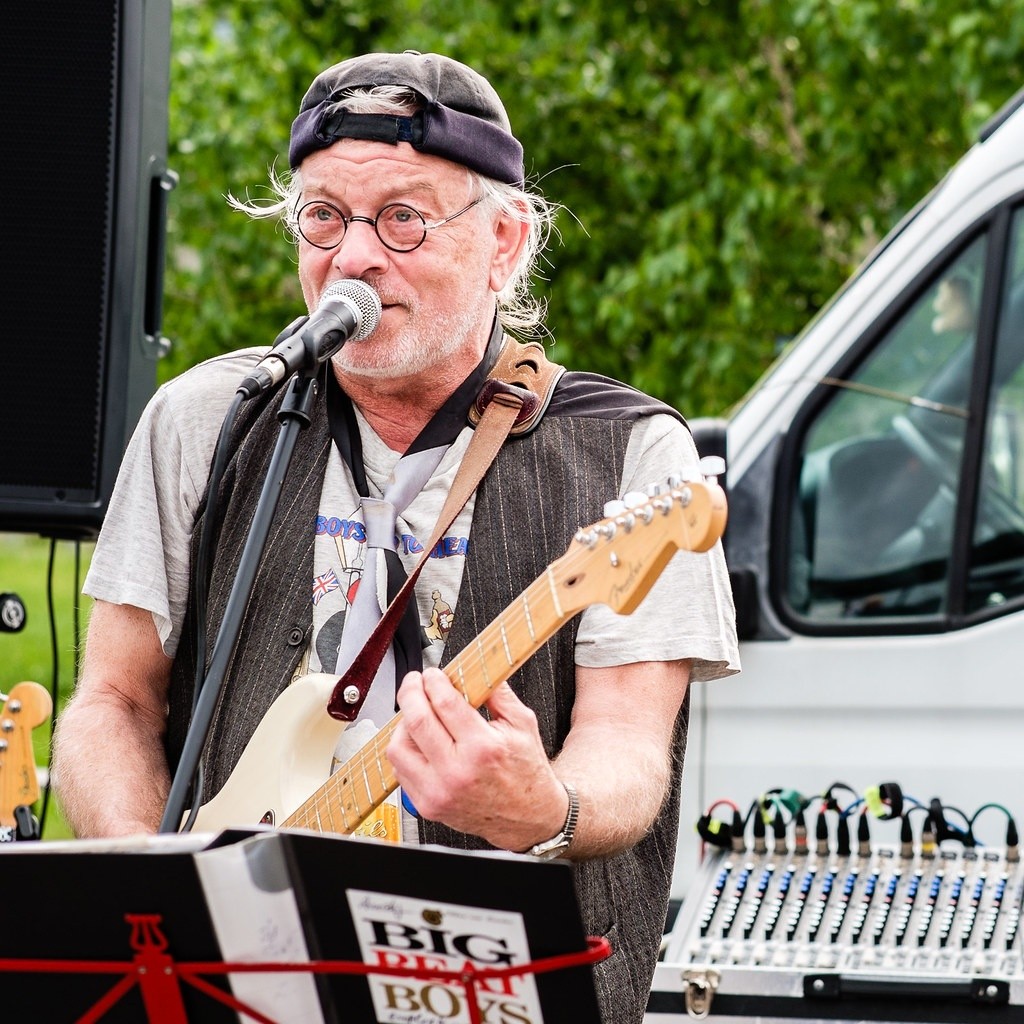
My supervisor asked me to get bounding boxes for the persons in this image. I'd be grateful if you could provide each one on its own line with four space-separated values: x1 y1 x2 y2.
50 50 740 1024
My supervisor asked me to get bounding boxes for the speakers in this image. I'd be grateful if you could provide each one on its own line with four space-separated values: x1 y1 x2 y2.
0 0 179 538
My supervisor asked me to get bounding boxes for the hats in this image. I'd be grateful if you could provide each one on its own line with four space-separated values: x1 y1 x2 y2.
288 49 524 192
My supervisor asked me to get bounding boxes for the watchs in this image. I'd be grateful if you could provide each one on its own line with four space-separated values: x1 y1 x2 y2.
523 780 578 859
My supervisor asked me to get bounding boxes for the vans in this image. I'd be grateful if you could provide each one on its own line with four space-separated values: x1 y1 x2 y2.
665 84 1024 909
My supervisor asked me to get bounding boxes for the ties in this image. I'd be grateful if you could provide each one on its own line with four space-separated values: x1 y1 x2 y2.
325 302 503 842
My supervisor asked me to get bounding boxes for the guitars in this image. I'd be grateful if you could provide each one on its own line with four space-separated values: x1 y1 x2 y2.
0 677 56 842
175 452 732 836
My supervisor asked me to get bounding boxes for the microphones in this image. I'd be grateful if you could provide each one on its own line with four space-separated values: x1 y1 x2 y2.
235 278 381 401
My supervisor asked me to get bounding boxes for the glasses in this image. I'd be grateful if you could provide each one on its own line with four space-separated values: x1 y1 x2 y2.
293 192 489 253
